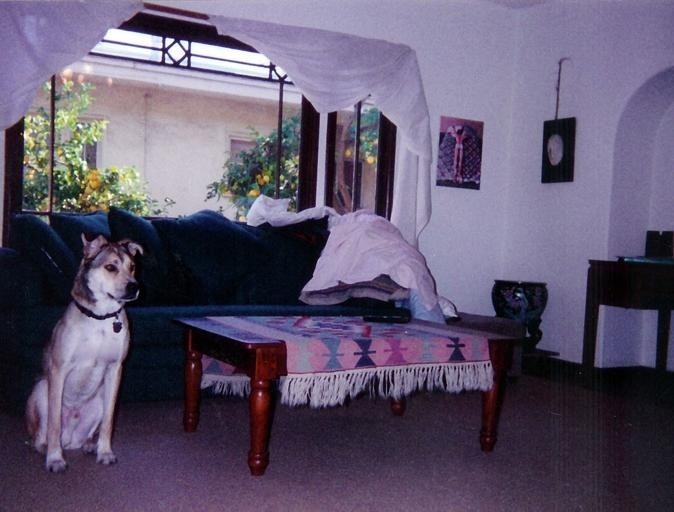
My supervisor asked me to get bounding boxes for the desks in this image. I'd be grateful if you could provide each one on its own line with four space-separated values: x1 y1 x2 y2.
581 254 674 385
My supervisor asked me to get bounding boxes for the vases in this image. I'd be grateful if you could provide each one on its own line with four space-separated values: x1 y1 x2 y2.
491 279 559 365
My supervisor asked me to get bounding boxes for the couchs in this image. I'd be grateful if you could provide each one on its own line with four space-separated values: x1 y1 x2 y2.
0 210 449 416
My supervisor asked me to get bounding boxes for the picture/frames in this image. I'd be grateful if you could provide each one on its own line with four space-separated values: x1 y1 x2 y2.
541 116 574 182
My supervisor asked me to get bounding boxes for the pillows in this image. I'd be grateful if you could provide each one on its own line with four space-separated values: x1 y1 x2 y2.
108 203 158 287
230 215 329 302
49 213 110 259
2 211 77 286
160 209 256 293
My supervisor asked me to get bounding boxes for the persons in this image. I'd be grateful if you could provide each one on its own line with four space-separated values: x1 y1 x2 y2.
451 124 466 183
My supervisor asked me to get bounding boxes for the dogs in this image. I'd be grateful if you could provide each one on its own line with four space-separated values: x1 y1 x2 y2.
22 230 145 475
